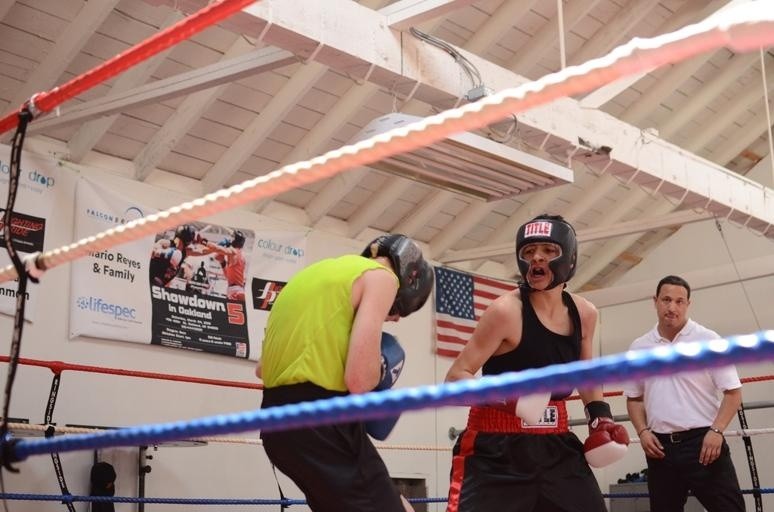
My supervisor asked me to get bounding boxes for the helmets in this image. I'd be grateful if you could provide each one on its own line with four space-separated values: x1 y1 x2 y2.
361 234 434 317
515 219 577 291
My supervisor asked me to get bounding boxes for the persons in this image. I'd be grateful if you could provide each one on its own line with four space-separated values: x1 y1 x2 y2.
256 234 434 512
441 215 628 512
152 224 246 300
623 274 746 512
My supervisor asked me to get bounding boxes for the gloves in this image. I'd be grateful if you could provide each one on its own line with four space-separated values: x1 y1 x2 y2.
583 416 630 468
364 416 399 441
486 390 552 426
371 332 405 392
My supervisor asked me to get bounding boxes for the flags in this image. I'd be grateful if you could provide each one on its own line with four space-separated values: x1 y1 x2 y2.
436 267 521 359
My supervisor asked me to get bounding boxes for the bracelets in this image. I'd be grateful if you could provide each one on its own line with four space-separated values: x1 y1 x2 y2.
636 428 650 438
709 427 723 436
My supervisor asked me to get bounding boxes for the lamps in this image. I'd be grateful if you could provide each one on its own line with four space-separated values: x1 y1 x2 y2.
342 91 577 206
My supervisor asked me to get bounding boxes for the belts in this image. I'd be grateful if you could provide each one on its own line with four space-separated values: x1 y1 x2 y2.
651 426 710 444
